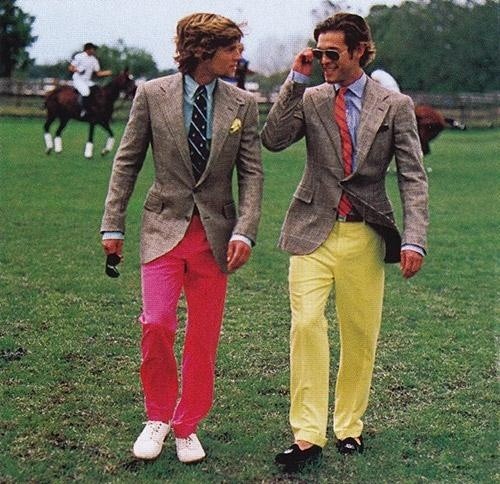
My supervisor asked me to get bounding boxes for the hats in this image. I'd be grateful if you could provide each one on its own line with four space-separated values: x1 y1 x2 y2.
83 43 100 50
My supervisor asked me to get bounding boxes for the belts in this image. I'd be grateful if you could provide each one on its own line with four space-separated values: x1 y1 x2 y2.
336 214 365 223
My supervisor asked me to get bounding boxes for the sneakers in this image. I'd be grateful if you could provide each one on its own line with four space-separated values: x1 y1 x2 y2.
132 421 171 459
275 442 323 463
342 435 364 456
176 432 207 463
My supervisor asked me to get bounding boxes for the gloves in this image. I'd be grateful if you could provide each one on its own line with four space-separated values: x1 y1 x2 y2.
79 68 86 75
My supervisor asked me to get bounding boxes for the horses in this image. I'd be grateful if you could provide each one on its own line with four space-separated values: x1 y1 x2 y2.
41 65 138 160
416 105 446 154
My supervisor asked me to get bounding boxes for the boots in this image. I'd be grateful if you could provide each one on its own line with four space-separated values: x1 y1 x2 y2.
79 96 89 117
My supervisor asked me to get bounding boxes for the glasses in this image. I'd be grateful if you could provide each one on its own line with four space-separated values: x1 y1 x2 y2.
312 43 349 62
105 253 123 278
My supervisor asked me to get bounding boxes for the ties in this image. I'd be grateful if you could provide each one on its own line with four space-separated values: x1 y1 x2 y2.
335 87 353 217
187 86 207 178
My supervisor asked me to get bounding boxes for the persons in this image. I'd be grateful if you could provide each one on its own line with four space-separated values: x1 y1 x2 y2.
67 42 112 119
257 11 431 466
98 12 267 465
